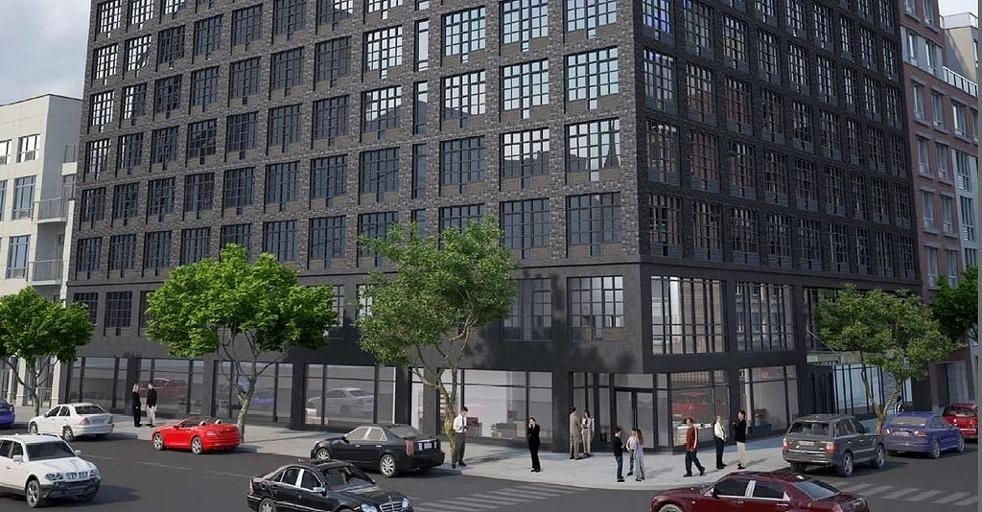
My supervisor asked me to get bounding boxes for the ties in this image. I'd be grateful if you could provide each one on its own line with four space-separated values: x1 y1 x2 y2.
462 418 465 434
720 425 727 441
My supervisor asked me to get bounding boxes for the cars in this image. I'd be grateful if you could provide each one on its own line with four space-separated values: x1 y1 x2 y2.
649 469 872 512
0 395 18 429
670 391 730 423
940 401 978 443
138 372 376 418
307 420 447 479
246 456 416 512
883 409 967 460
27 402 116 445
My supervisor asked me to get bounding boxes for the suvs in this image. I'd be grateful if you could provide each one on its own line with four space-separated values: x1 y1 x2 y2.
0 429 101 509
781 412 891 477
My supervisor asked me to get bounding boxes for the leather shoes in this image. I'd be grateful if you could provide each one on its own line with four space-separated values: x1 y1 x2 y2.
452 462 466 469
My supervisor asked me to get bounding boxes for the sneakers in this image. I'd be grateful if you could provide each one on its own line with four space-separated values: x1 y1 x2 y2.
737 463 746 471
683 466 706 478
531 467 541 473
616 472 646 483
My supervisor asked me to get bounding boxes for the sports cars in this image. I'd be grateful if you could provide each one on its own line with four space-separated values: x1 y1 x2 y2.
149 416 242 458
